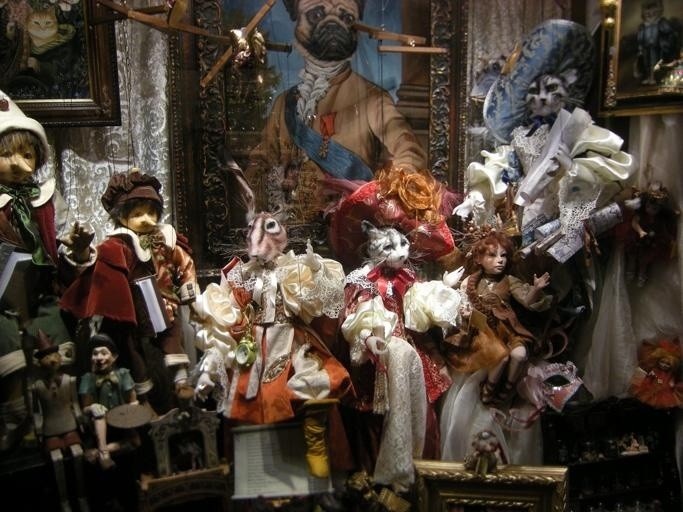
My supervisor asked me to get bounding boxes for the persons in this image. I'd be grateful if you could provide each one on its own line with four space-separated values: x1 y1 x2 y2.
1 2 682 507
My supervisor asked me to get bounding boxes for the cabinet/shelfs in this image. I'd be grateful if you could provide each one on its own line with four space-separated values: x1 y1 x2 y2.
540 395 683 512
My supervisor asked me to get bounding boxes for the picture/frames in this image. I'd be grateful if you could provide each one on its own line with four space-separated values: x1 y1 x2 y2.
596 0 683 118
412 460 568 512
149 404 221 477
136 463 244 512
0 0 121 131
164 0 467 292
